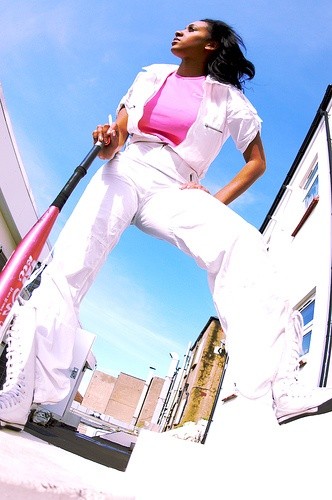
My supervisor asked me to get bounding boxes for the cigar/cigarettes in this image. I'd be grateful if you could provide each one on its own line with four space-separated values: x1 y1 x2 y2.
106 111 116 137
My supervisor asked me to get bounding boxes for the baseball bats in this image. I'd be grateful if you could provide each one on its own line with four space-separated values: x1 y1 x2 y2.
0 131 111 331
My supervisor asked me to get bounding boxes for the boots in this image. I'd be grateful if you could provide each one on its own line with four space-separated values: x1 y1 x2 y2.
0 304 38 432
270 307 332 424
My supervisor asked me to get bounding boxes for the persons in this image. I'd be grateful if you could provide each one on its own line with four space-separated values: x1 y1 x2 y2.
1 18 332 432
